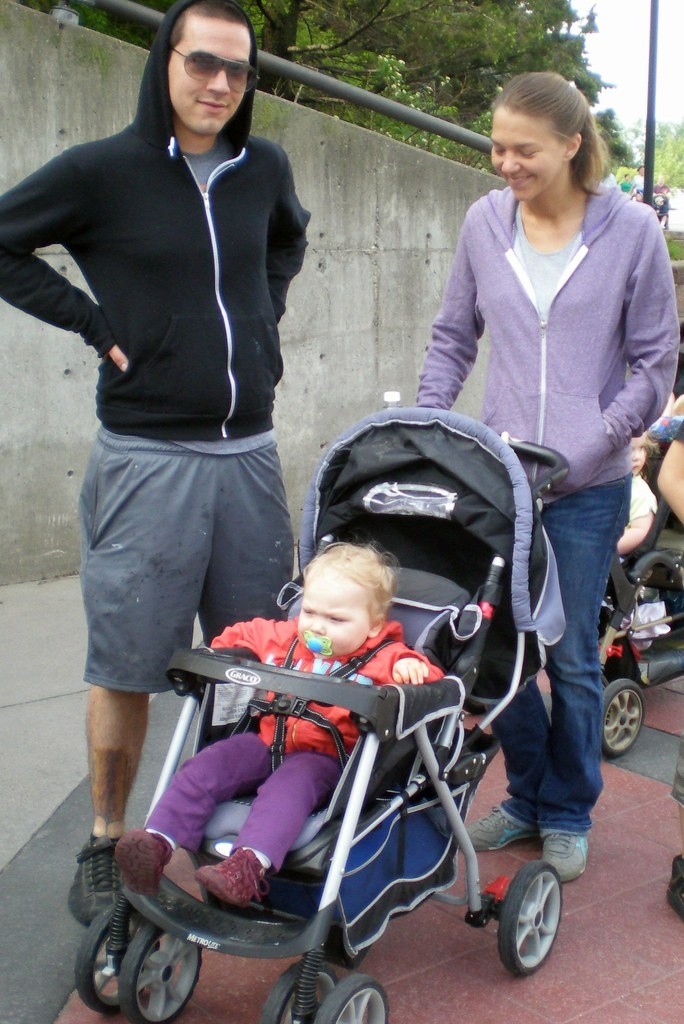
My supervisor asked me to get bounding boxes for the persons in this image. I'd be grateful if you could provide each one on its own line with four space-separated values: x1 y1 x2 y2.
417 71 684 922
117 542 444 906
0 0 311 926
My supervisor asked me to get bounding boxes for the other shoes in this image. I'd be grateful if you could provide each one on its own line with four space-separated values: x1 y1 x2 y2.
667 855 684 918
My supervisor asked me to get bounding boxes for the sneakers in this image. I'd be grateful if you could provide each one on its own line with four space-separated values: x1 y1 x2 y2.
193 848 270 908
463 805 539 850
67 833 123 926
543 831 588 881
114 828 172 895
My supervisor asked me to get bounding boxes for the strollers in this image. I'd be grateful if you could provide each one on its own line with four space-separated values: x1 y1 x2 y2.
73 408 571 1024
462 447 684 757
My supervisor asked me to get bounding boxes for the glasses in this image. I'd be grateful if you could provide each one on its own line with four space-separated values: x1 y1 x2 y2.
169 45 261 93
